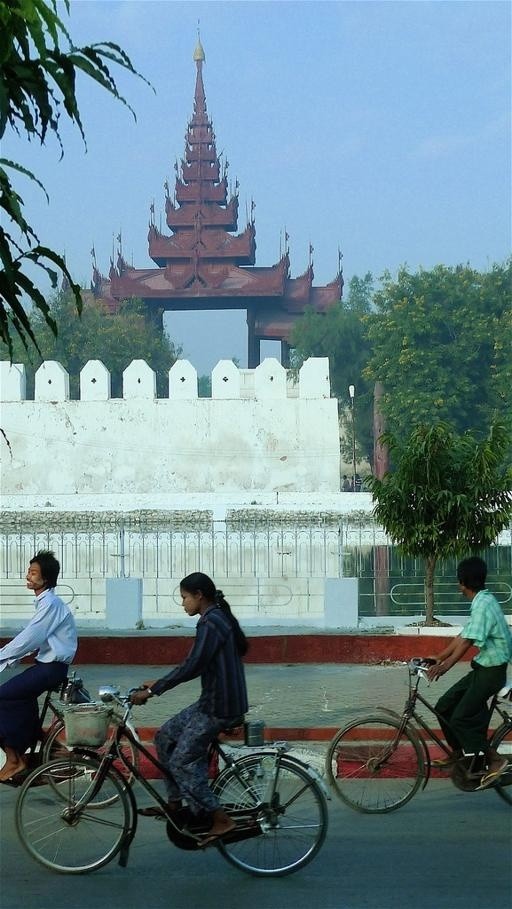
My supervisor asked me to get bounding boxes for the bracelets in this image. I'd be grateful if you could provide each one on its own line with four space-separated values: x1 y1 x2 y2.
146 687 154 697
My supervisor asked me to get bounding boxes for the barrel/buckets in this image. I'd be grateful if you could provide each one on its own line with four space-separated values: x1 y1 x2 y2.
244 718 265 746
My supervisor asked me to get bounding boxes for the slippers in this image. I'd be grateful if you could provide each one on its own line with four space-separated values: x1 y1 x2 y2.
474 759 509 792
137 804 182 817
197 821 236 847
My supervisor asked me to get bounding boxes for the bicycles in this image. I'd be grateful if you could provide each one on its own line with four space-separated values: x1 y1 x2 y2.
323 656 512 815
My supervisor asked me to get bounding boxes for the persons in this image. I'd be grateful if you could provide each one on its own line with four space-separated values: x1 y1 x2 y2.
129 571 250 847
422 557 512 791
0 549 77 781
